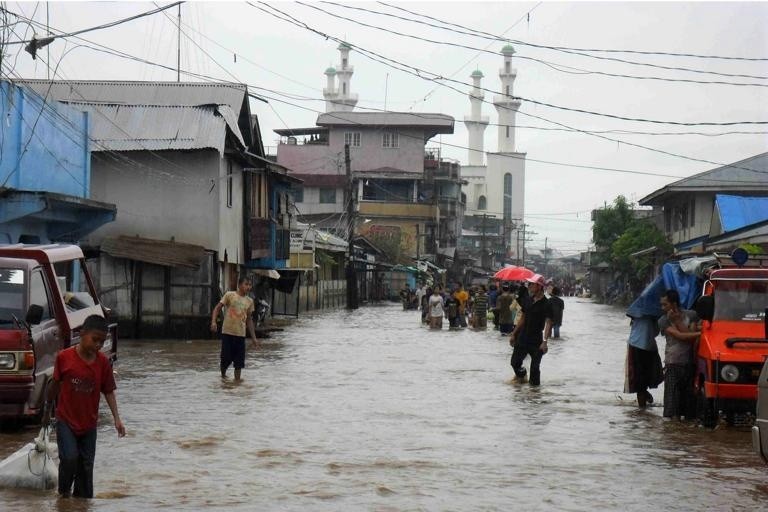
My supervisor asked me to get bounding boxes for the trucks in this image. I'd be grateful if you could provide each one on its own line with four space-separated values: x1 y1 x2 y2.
751 357 767 462
679 247 766 430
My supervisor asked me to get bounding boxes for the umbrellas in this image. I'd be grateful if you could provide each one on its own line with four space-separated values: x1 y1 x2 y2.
494 267 536 298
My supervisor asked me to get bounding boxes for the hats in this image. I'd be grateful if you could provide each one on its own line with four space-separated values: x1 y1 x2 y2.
526 274 545 286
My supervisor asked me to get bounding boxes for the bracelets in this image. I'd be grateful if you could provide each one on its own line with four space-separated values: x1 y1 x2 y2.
543 339 547 342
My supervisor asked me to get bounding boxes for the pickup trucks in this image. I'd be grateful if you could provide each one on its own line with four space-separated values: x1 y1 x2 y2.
0 242 118 434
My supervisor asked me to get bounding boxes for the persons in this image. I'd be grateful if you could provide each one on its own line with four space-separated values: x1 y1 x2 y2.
627 318 660 408
41 314 126 499
401 278 583 339
510 274 553 385
211 276 260 381
658 289 702 421
